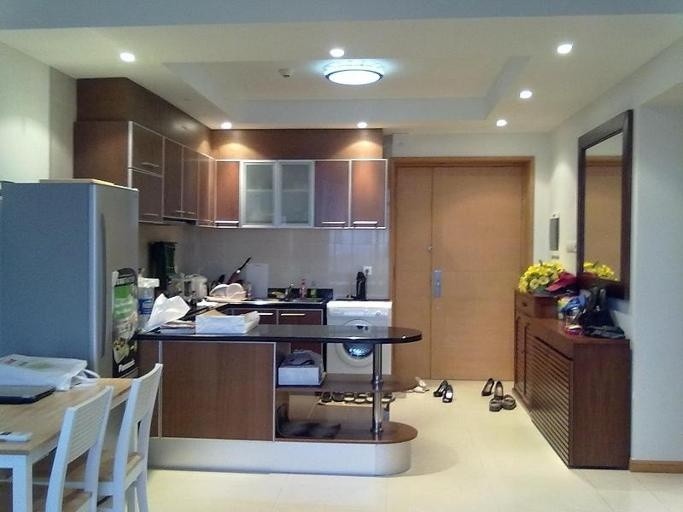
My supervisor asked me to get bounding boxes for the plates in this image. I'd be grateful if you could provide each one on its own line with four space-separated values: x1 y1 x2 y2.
226 283 243 297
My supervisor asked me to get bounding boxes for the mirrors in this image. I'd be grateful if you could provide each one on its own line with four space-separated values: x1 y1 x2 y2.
576 108 632 302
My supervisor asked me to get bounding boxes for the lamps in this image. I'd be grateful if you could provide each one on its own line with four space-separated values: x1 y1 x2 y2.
322 63 386 87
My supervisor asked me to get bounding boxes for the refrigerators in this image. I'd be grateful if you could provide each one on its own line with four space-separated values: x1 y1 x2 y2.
0 181 140 384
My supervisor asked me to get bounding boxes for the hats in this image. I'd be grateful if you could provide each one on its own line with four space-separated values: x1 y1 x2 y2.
545 272 577 292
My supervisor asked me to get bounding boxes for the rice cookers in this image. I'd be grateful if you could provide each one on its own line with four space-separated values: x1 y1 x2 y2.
187 273 207 298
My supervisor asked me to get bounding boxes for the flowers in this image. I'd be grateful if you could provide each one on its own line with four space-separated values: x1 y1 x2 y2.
581 258 616 281
517 261 563 293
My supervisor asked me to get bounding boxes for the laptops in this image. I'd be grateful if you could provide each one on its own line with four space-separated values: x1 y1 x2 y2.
0 385 56 405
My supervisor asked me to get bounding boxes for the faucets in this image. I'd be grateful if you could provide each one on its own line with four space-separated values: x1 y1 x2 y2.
271 291 285 299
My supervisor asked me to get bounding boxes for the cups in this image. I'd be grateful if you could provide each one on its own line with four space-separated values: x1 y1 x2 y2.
557 300 568 320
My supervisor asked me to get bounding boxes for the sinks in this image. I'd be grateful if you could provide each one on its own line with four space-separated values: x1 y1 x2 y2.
244 298 276 305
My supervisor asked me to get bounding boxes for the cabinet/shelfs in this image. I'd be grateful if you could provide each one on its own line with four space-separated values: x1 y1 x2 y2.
74 120 164 224
214 160 237 229
194 153 214 228
163 135 196 224
232 309 325 370
513 313 632 469
239 159 316 229
310 159 389 231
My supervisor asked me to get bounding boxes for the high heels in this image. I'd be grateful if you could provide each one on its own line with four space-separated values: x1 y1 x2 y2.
434 380 453 402
482 378 494 396
494 381 503 400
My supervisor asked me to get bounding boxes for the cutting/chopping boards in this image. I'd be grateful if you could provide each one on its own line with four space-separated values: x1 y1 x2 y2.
245 263 269 299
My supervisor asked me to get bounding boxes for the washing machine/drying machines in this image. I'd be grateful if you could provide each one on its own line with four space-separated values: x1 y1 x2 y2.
325 298 392 376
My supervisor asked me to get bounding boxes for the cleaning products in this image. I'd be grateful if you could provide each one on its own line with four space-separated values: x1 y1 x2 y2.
299 279 307 301
310 282 317 298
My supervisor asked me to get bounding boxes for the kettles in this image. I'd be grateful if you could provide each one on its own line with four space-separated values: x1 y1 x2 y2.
354 272 366 300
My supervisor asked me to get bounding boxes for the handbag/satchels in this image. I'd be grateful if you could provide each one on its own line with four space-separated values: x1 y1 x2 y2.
561 284 625 340
0 353 100 391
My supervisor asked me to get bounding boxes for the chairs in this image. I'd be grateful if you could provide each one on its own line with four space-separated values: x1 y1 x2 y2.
28 362 164 511
0 386 113 511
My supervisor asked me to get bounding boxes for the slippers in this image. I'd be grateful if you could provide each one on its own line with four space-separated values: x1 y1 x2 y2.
490 395 516 411
321 392 394 403
412 377 429 392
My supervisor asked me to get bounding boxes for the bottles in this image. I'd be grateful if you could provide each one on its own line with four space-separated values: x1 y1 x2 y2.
283 288 289 301
298 279 307 298
183 275 191 296
138 279 154 329
309 281 316 298
